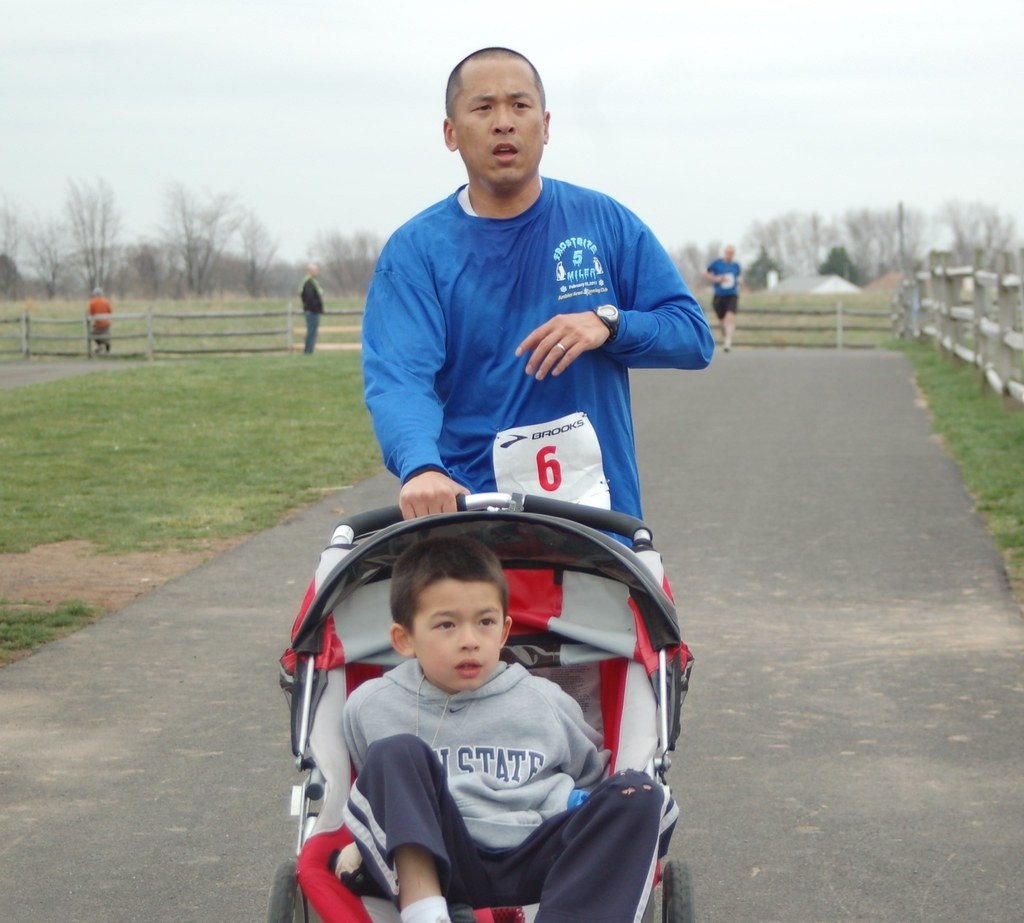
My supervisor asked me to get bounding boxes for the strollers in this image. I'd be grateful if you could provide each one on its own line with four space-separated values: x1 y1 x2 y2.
264 488 708 923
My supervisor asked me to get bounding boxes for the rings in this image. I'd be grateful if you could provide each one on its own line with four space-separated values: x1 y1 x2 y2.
558 343 565 351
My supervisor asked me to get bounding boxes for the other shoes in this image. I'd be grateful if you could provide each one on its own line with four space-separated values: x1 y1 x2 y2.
95 344 104 354
723 345 731 353
106 343 109 352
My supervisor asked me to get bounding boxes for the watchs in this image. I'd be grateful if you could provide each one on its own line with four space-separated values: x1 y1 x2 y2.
594 304 620 340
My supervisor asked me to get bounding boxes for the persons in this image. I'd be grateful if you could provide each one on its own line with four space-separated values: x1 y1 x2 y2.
362 46 713 548
344 536 679 922
302 266 325 353
706 244 741 351
88 287 112 354
